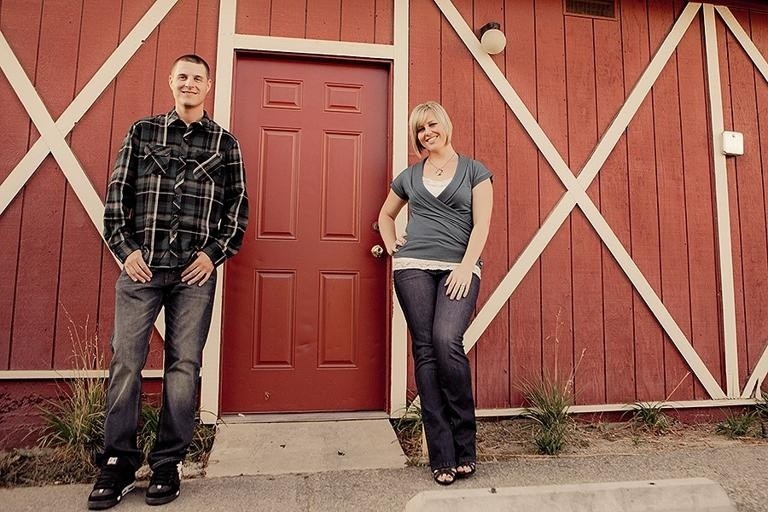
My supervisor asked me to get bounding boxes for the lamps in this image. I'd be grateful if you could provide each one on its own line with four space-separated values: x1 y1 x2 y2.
479 21 508 55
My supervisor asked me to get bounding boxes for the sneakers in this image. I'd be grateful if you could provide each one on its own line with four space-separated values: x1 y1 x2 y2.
146 461 184 505
87 454 134 508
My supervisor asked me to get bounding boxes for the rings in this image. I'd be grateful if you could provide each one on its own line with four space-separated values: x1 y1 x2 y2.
460 284 466 286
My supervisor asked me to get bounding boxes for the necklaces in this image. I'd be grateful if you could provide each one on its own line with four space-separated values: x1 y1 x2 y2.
428 151 456 176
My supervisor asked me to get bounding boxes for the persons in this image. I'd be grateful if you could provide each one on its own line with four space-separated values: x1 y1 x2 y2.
376 100 495 485
86 53 248 509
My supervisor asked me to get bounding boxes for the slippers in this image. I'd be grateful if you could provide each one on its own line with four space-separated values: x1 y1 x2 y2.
433 467 456 485
457 461 477 477
